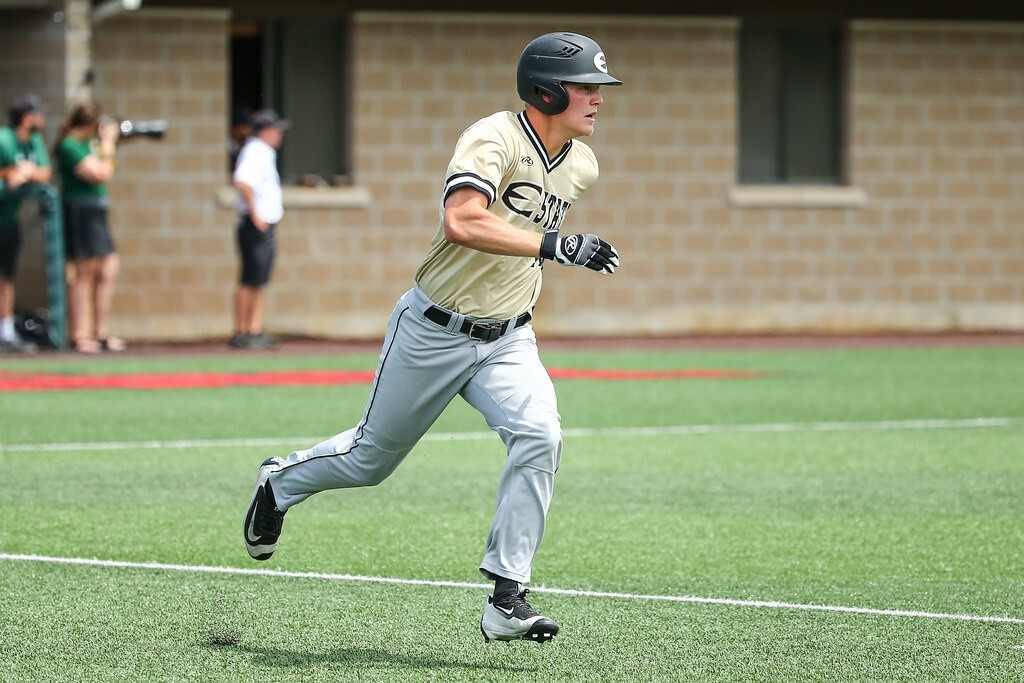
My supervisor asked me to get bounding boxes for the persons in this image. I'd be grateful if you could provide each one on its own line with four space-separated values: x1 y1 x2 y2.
51 98 127 354
229 104 252 170
0 96 50 354
228 109 292 351
245 32 624 645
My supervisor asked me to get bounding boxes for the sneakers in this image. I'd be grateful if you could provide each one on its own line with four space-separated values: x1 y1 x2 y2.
480 582 559 644
243 456 288 561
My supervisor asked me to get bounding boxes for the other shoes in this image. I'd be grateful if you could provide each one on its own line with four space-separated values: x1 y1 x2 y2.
230 331 282 350
68 336 126 353
0 334 39 356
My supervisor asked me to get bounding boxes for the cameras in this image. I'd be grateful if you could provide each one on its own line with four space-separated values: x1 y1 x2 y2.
115 118 167 140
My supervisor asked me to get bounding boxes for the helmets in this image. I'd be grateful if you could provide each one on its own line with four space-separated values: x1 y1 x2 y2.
516 31 624 115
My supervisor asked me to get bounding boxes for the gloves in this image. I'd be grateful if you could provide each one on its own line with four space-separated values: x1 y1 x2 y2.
539 228 621 274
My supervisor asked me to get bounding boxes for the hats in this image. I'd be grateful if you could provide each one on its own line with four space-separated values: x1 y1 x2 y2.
251 110 292 132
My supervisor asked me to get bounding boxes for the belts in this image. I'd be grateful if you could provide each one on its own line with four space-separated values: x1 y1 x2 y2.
424 306 534 341
